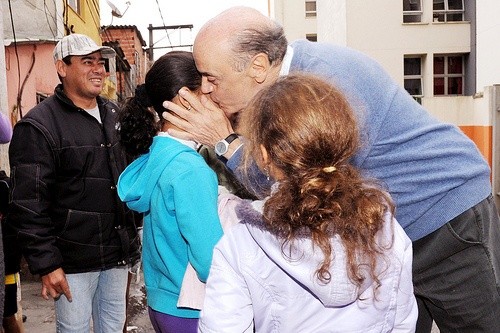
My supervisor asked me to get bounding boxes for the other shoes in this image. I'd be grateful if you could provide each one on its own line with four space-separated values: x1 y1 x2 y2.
22 315 27 322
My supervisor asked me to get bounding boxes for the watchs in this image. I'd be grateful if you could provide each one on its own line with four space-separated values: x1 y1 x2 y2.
214 132 240 164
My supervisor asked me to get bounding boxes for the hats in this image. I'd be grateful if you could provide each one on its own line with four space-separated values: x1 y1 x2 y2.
53 34 116 65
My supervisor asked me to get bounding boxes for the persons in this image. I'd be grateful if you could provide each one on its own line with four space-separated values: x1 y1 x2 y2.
162 6 500 333
196 70 419 333
0 33 143 333
116 50 227 333
